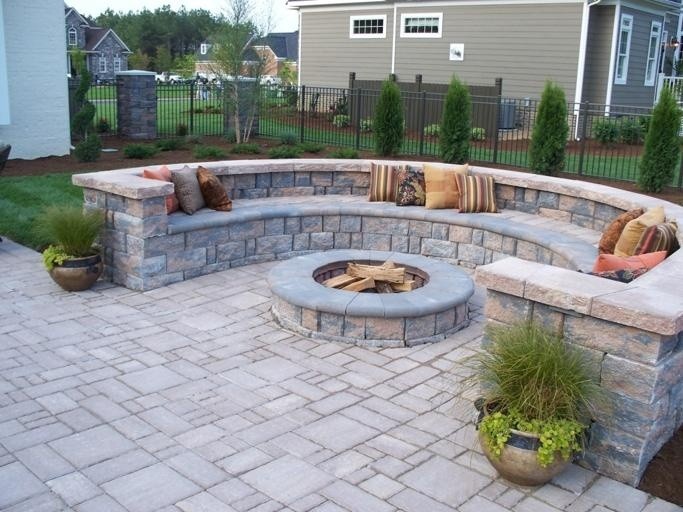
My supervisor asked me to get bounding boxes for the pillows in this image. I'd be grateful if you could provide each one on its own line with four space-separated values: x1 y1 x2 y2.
363 163 505 223
137 163 231 225
584 200 679 287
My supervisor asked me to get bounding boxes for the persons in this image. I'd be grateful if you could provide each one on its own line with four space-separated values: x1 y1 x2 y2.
200 78 210 101
194 78 203 99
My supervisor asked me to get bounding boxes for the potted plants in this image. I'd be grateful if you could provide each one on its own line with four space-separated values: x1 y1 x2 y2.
30 201 118 295
465 320 603 488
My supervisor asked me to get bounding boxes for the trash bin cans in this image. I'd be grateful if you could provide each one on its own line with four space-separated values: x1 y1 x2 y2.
499 99 516 129
202 90 207 100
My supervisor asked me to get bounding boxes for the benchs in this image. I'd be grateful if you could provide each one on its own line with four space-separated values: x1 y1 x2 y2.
71 159 683 488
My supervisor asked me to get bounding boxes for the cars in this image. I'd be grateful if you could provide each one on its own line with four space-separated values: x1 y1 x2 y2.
154 70 277 87
